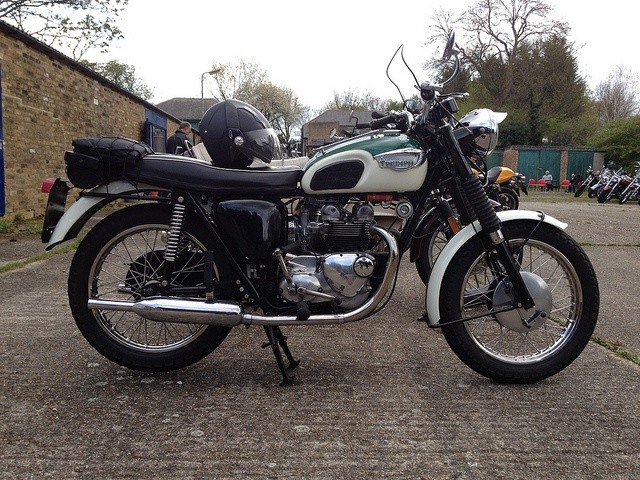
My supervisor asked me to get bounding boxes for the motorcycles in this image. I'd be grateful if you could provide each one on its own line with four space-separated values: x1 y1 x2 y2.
574 165 640 204
472 167 528 212
39 33 599 387
395 186 467 287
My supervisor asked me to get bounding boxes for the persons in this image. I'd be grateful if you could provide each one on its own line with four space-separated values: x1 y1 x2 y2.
568 174 578 193
165 122 193 152
542 169 553 192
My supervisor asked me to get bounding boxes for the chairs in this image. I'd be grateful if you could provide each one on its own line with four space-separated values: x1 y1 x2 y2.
528 179 536 191
537 180 546 192
552 180 559 192
560 180 571 192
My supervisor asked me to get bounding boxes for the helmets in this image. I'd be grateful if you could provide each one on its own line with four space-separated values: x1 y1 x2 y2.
197 99 283 169
